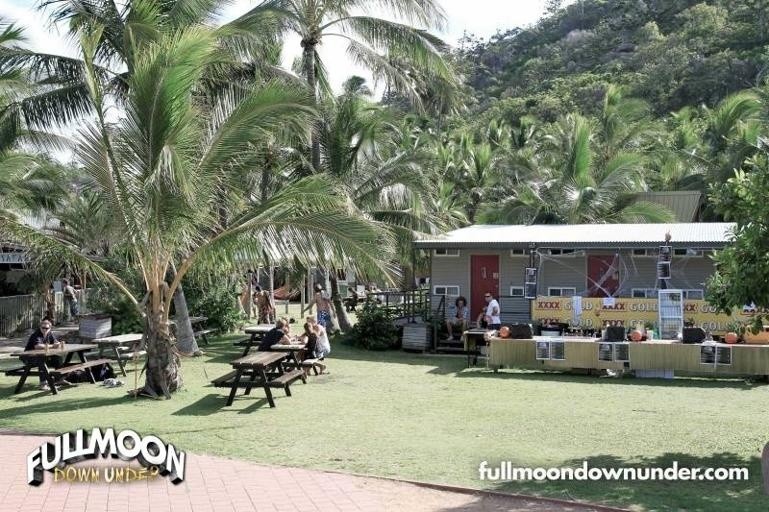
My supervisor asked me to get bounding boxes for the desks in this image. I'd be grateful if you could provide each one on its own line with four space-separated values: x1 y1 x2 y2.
189 315 278 357
463 327 769 375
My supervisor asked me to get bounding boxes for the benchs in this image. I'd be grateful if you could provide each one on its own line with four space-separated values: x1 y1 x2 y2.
0 328 320 407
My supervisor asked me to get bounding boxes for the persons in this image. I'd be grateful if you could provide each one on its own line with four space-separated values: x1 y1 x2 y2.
373 286 383 303
345 287 359 310
61 278 82 324
446 291 502 341
253 276 337 375
22 320 72 390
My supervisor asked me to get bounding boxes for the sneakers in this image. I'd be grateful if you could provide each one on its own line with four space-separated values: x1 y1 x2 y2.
446 336 453 340
57 380 69 385
41 385 48 390
461 336 464 341
319 364 326 375
